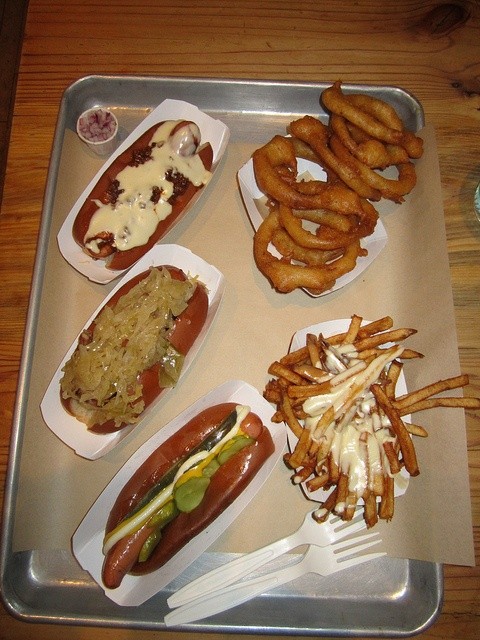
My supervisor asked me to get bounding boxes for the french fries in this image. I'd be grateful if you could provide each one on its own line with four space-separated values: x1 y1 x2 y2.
264 315 480 527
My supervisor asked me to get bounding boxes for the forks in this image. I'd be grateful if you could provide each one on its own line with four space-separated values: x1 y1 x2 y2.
167 494 370 610
163 518 389 629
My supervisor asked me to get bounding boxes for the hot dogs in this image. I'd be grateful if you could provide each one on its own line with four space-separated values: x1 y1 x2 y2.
102 403 275 585
73 119 213 270
59 262 208 432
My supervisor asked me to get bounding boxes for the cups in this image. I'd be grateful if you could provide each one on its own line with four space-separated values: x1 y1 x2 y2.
75 108 120 155
